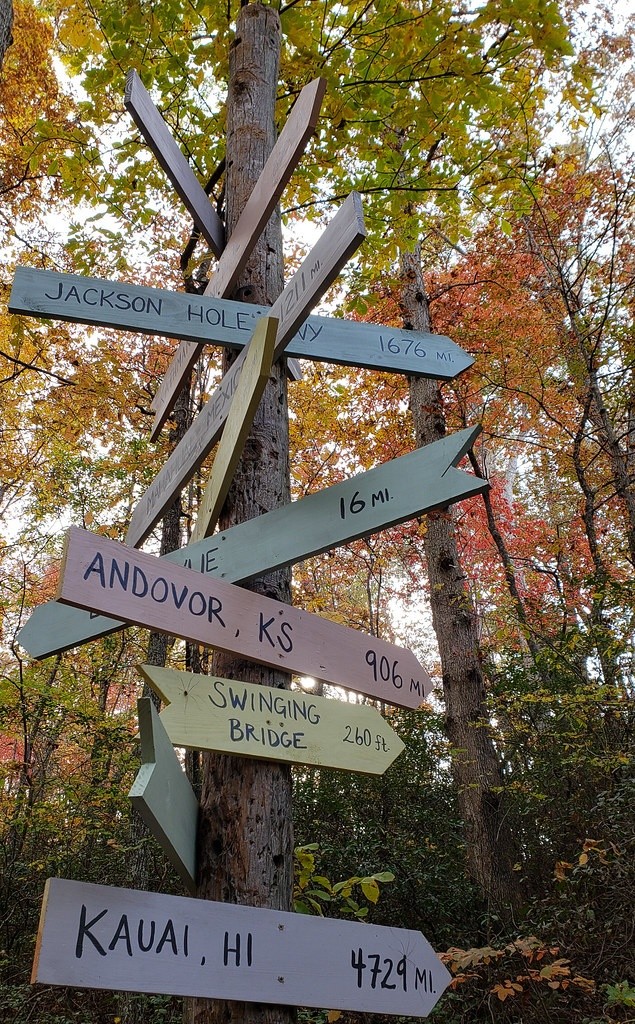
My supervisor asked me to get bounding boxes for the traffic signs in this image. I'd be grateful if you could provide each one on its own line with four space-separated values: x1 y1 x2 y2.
7 187 492 1018
